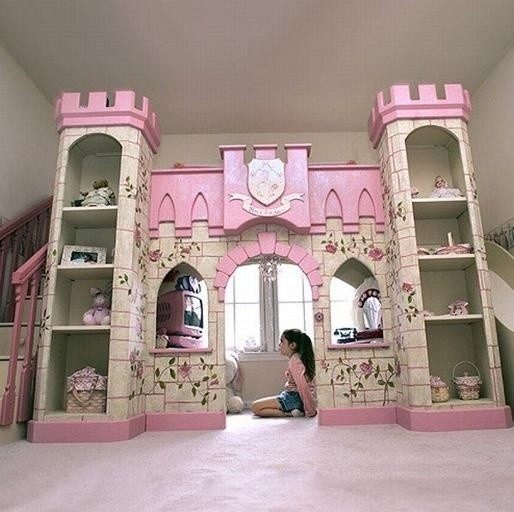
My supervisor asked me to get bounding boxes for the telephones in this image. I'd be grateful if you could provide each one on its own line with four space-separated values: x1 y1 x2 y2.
334 328 357 344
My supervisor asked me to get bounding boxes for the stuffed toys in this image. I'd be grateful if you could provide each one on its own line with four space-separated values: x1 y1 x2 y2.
225 351 244 414
79 179 115 206
82 281 112 325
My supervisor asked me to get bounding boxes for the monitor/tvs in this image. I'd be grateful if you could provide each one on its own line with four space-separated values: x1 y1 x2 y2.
157 289 204 339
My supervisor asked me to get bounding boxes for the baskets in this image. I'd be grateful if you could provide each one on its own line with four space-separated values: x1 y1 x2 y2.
452 360 482 400
435 243 471 254
157 335 167 347
431 377 449 402
65 376 108 413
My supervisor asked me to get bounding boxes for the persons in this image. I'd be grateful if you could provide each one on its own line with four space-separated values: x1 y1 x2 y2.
249 330 317 418
